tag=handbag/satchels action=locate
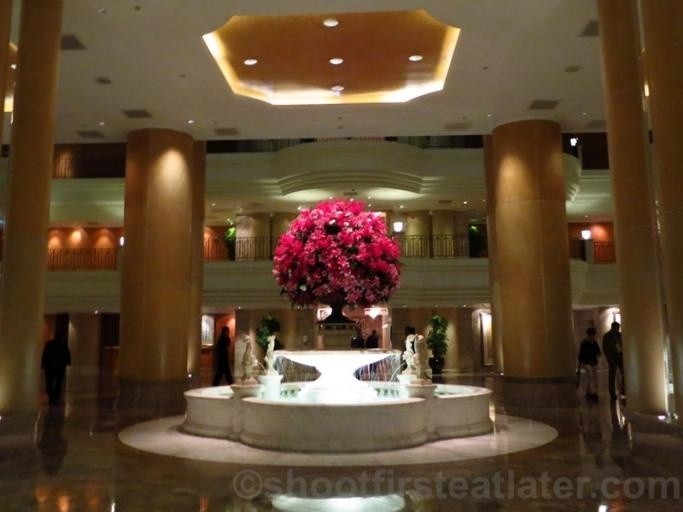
[572,371,581,388]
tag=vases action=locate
[314,302,357,349]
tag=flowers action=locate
[269,198,398,312]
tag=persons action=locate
[577,327,602,401]
[403,327,416,370]
[40,325,72,406]
[601,320,626,402]
[210,325,233,387]
[263,330,285,356]
[350,327,379,348]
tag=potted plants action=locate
[425,313,448,375]
